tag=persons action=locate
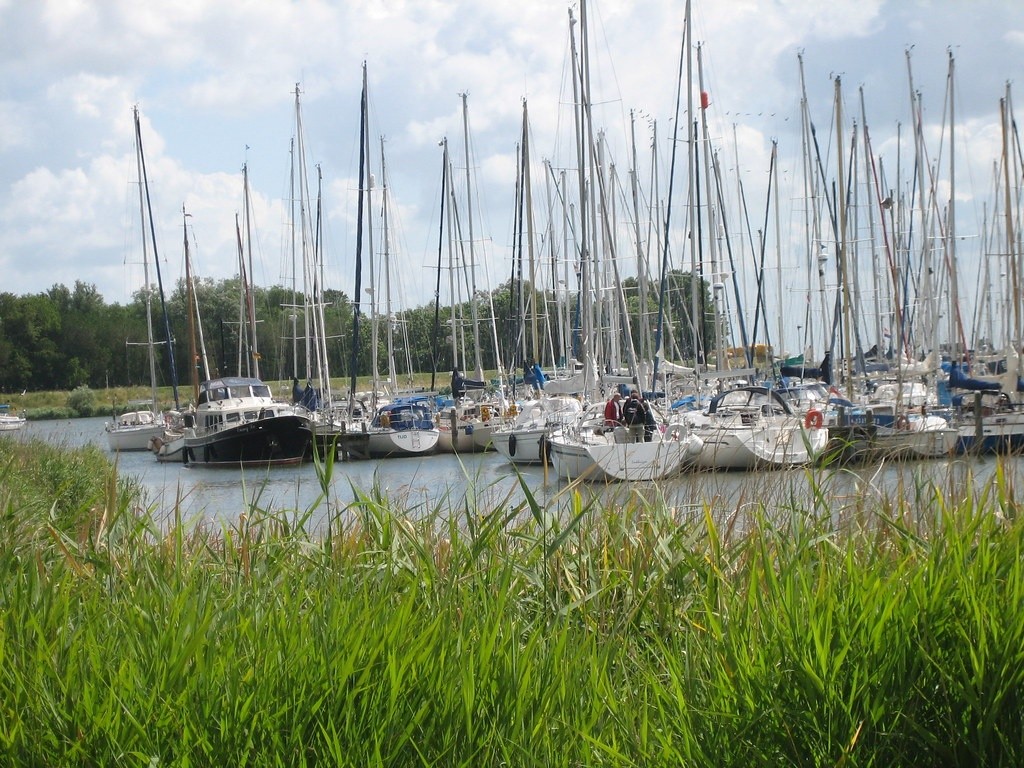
[604,393,623,427]
[623,389,657,444]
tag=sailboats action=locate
[103,0,1024,483]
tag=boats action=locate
[0,403,28,431]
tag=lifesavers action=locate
[804,410,822,430]
